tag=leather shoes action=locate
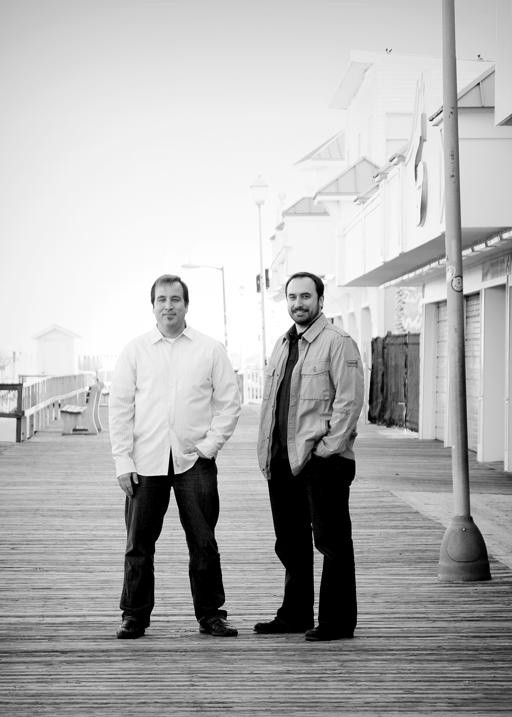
[304,624,354,641]
[199,617,238,637]
[116,618,148,639]
[253,619,314,634]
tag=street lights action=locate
[248,169,270,395]
[179,263,228,361]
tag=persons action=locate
[250,271,366,643]
[104,272,242,641]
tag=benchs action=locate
[58,381,104,437]
